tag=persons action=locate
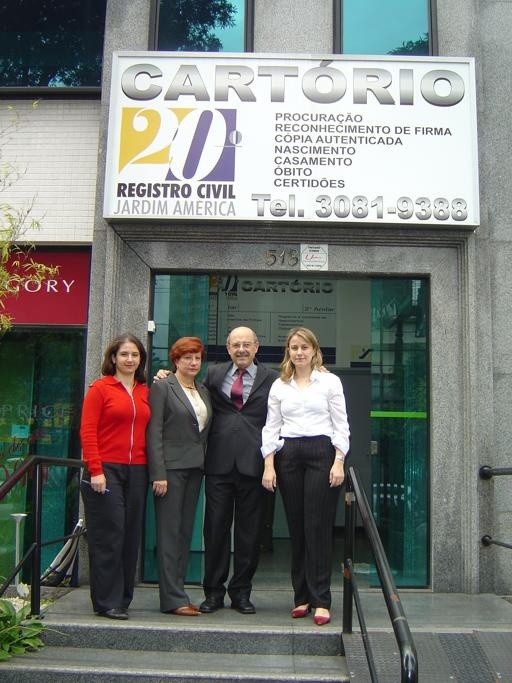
[260,324,352,626]
[152,324,331,616]
[77,331,152,620]
[145,335,214,617]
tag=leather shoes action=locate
[292,604,330,625]
[174,597,224,615]
[231,599,255,614]
[107,608,128,619]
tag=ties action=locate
[231,369,246,410]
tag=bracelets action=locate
[335,455,344,462]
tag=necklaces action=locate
[179,378,196,389]
